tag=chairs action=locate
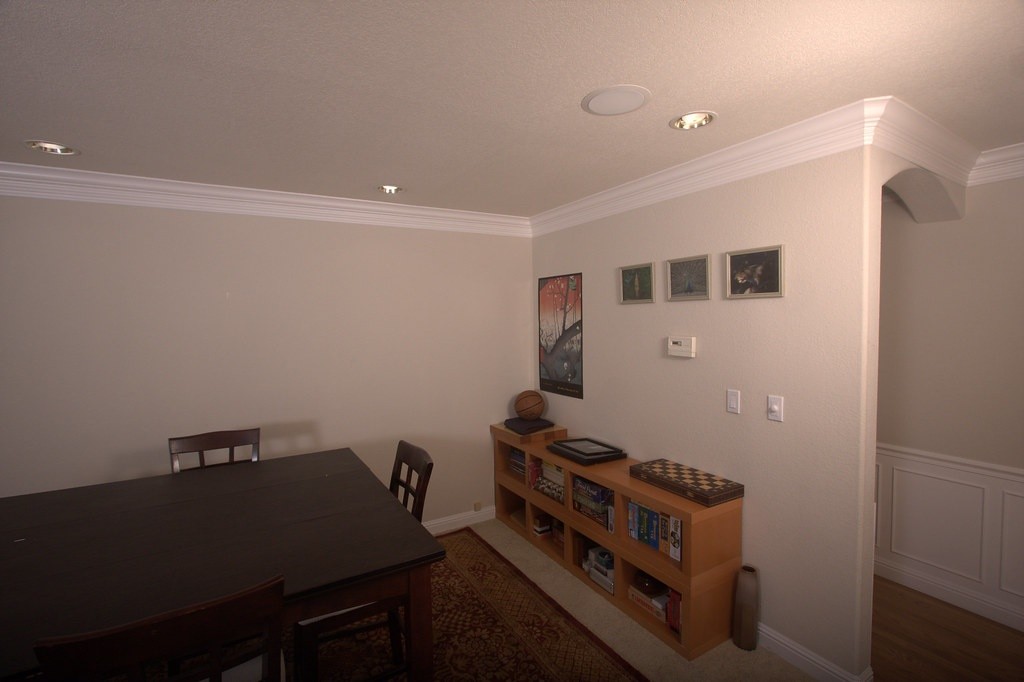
[33,574,287,682]
[168,428,261,473]
[295,442,433,682]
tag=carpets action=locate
[280,525,650,682]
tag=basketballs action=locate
[514,390,545,421]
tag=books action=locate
[627,576,681,636]
[573,535,615,595]
[529,459,564,502]
[624,497,682,561]
[572,476,613,528]
[533,516,564,549]
[510,447,525,475]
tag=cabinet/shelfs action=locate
[490,420,745,661]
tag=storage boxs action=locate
[628,503,639,540]
[648,510,659,550]
[638,507,650,545]
[659,511,671,556]
[589,568,614,595]
[670,516,682,562]
[628,583,668,624]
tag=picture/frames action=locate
[725,243,786,299]
[538,272,584,400]
[667,253,713,301]
[618,262,656,304]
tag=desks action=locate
[0,447,447,682]
